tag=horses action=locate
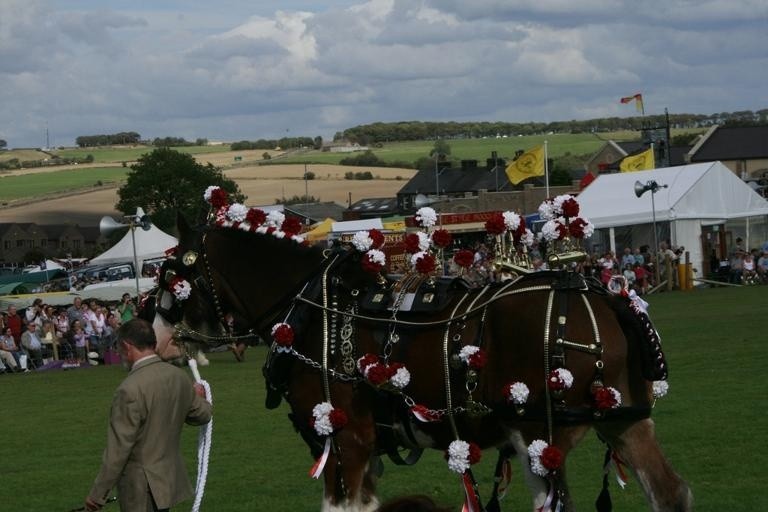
[149,210,693,512]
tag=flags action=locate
[619,148,654,173]
[505,145,546,186]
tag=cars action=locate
[0,265,41,275]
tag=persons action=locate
[0,264,251,373]
[84,318,213,512]
